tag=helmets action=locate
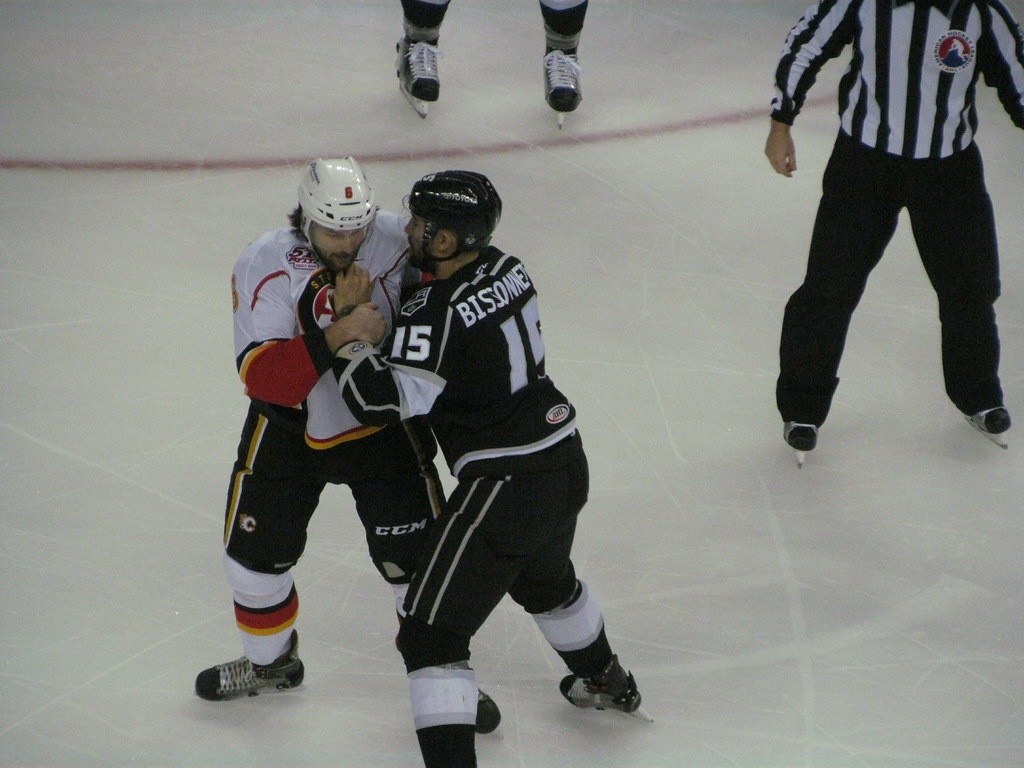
[296,153,377,228]
[410,170,504,235]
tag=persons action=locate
[396,0,588,130]
[196,156,654,768]
[765,0,1024,469]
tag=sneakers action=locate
[542,50,583,131]
[783,422,818,466]
[397,36,441,120]
[476,690,502,736]
[965,407,1011,449]
[559,654,654,723]
[194,628,306,702]
